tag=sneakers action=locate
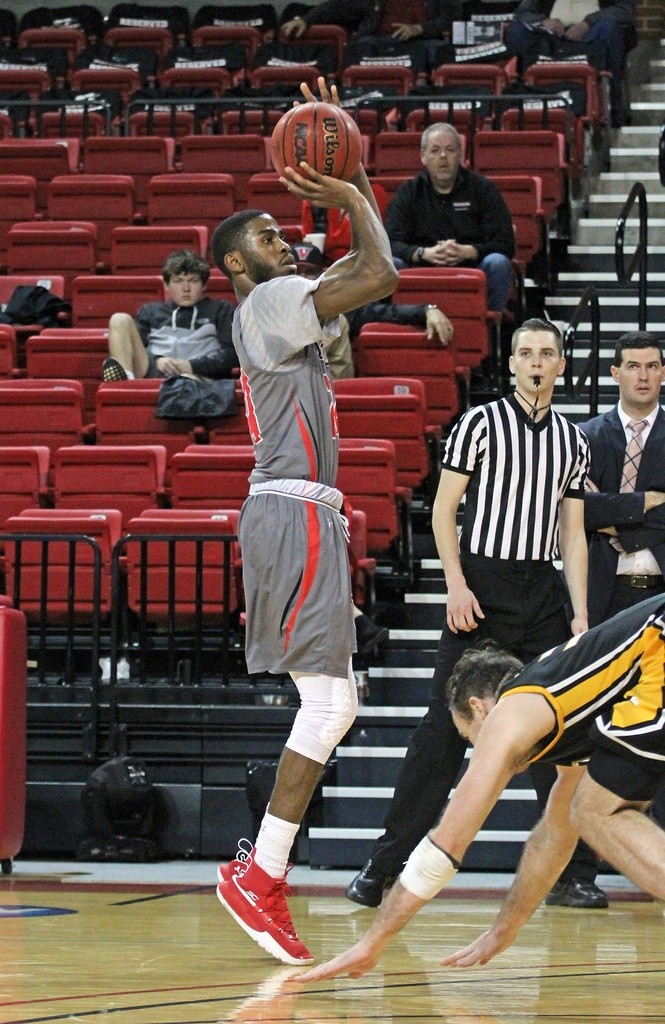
[102,358,129,383]
[214,859,315,968]
[217,846,259,880]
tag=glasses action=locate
[293,268,319,275]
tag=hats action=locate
[290,243,324,270]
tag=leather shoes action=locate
[544,877,610,908]
[345,859,385,908]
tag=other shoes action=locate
[354,613,389,655]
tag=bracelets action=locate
[425,304,438,312]
[418,246,424,261]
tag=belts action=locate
[615,574,664,590]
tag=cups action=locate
[305,233,326,255]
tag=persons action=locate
[212,156,400,968]
[385,122,516,313]
[102,248,240,383]
[572,332,665,633]
[288,242,455,379]
[345,314,611,908]
[277,0,637,86]
[292,593,665,983]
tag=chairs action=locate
[0,0,611,674]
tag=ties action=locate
[619,418,650,495]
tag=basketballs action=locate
[269,100,362,189]
